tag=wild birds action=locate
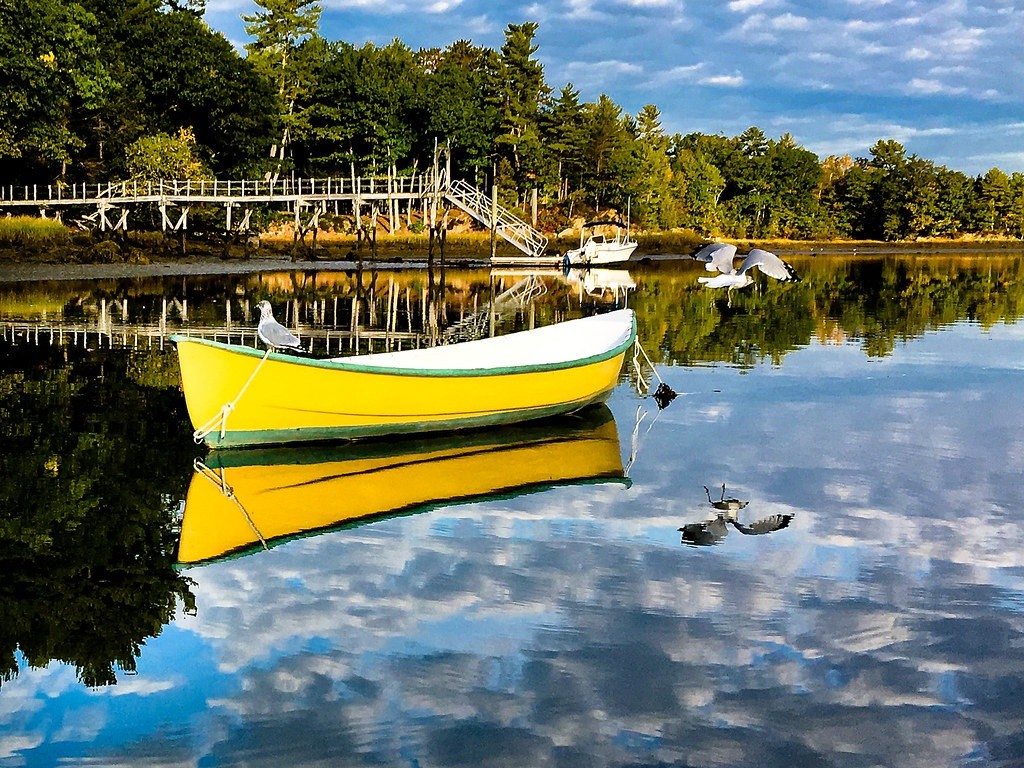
[254,299,308,354]
[675,482,798,549]
[689,240,804,312]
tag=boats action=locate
[169,303,639,450]
[566,194,639,266]
[567,270,635,311]
[173,403,634,576]
